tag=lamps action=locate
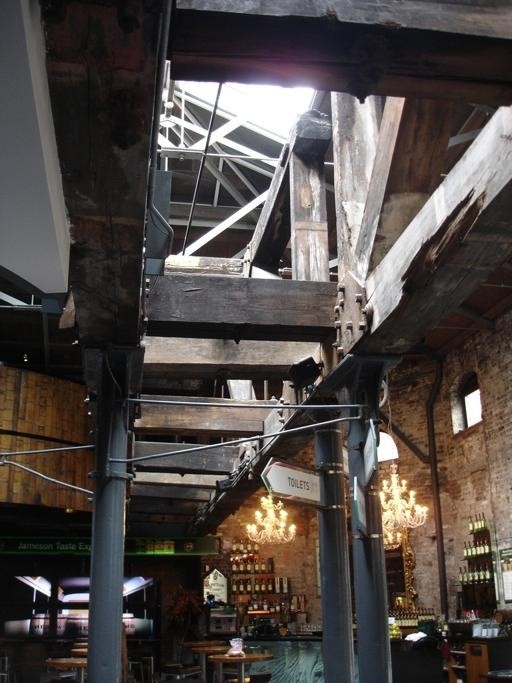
[246,492,297,546]
[378,372,429,551]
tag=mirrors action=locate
[382,524,418,611]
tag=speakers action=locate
[289,356,321,391]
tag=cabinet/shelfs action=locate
[229,551,294,615]
[450,529,493,587]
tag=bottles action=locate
[231,577,274,594]
[231,534,259,553]
[231,556,267,574]
[388,605,437,629]
[463,537,491,558]
[468,511,487,533]
[458,563,493,584]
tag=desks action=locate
[42,633,276,683]
